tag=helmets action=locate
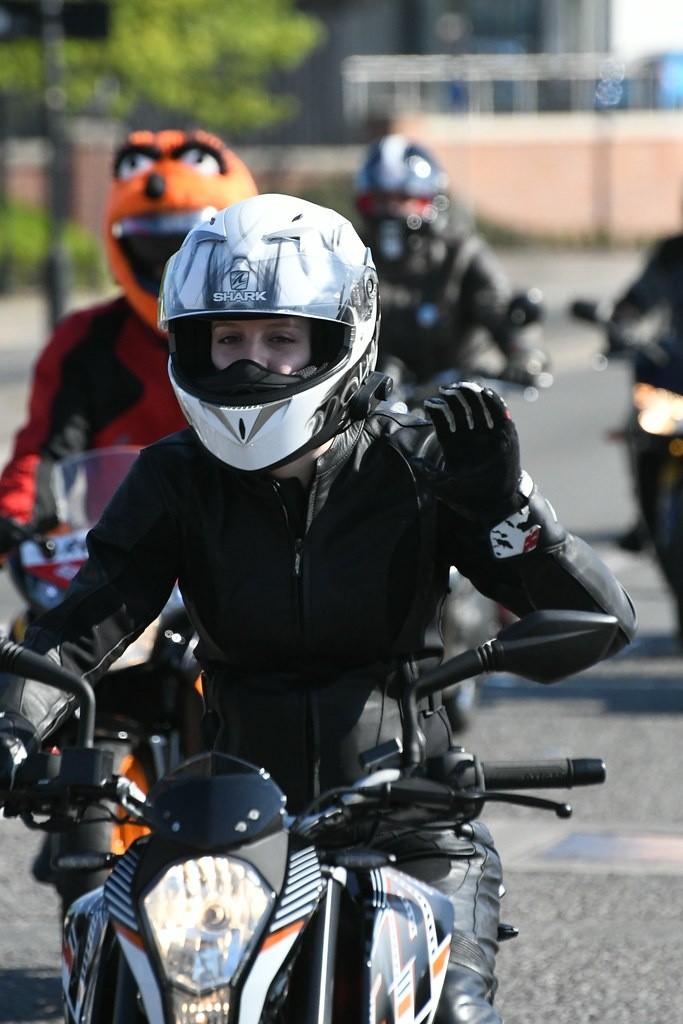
[156,194,393,477]
[354,133,448,285]
[112,215,210,295]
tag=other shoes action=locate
[618,520,651,551]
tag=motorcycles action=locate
[351,289,549,730]
[1,447,215,799]
[2,543,635,1024]
[565,293,683,638]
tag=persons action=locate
[606,234,683,551]
[0,130,258,617]
[352,135,548,625]
[0,193,636,1024]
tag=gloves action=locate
[0,710,41,808]
[499,355,533,394]
[409,384,566,529]
